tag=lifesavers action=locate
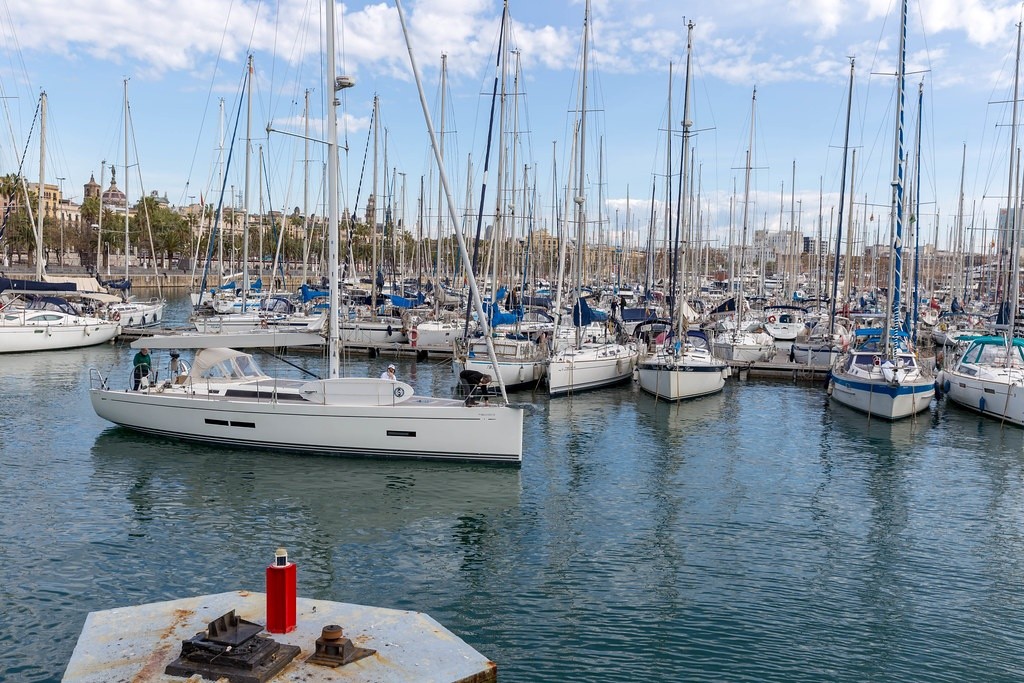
[768,315,776,323]
[112,310,122,322]
[261,319,267,326]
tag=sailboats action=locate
[937,169,1024,425]
[88,0,522,471]
[828,0,937,421]
[638,16,732,400]
[543,0,647,394]
[452,0,549,391]
[0,0,1024,373]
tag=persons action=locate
[380,364,396,381]
[132,347,154,391]
[460,370,493,407]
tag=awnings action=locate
[0,274,124,305]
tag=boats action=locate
[0,291,122,353]
